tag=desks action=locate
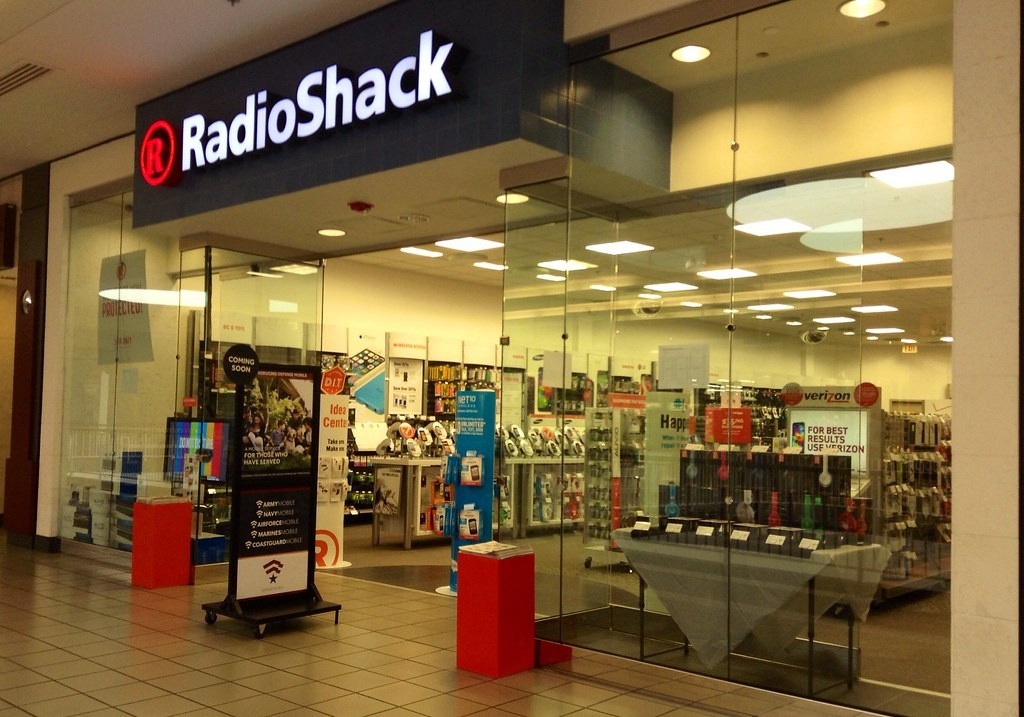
[610,527,893,697]
[369,457,585,551]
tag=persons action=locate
[241,409,312,455]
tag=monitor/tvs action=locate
[163,417,234,486]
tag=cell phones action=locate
[349,346,387,395]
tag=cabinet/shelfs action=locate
[344,451,377,525]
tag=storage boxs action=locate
[192,532,225,564]
[59,473,171,552]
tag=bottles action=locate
[686,451,698,478]
[840,498,856,531]
[819,454,832,487]
[718,451,729,479]
[768,491,781,526]
[856,504,868,537]
[801,495,814,529]
[665,484,680,517]
